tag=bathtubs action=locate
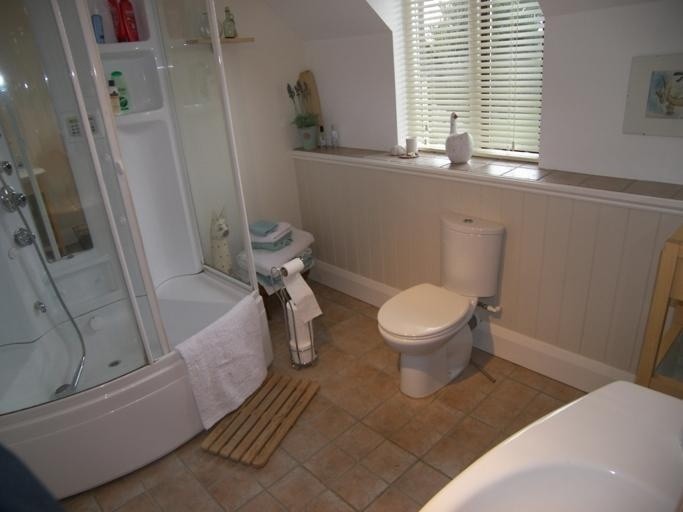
[421,380,682,512]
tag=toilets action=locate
[377,213,505,398]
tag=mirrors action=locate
[0,0,96,263]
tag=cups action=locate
[406,139,418,153]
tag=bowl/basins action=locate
[391,145,405,155]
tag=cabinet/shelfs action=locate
[635,224,683,399]
[79,0,169,129]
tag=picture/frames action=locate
[620,53,683,136]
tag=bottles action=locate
[91,9,104,42]
[223,5,237,38]
[319,124,337,149]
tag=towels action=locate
[249,219,316,297]
[176,295,267,430]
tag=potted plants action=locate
[287,81,322,151]
